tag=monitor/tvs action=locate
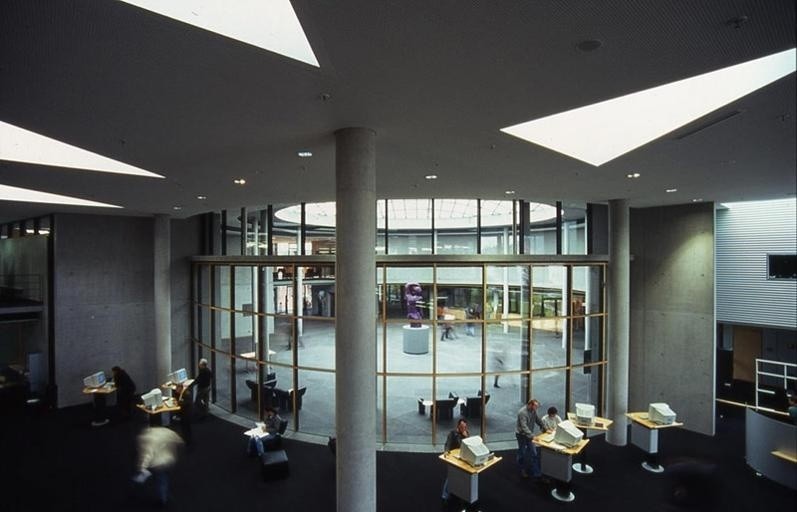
[555,419,585,448]
[141,387,164,409]
[459,435,489,467]
[648,402,677,424]
[167,367,189,384]
[83,370,107,388]
[575,402,595,424]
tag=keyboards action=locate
[640,415,648,419]
[164,400,175,408]
[541,431,556,442]
[453,454,461,459]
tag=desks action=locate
[438,411,685,505]
[81,378,185,427]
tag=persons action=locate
[438,418,470,507]
[438,305,452,340]
[464,306,476,337]
[513,399,546,480]
[110,365,136,419]
[243,406,283,457]
[191,358,212,408]
[404,281,425,328]
[136,422,186,506]
[540,406,561,432]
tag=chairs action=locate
[419,390,490,423]
[245,377,306,413]
[263,418,288,449]
